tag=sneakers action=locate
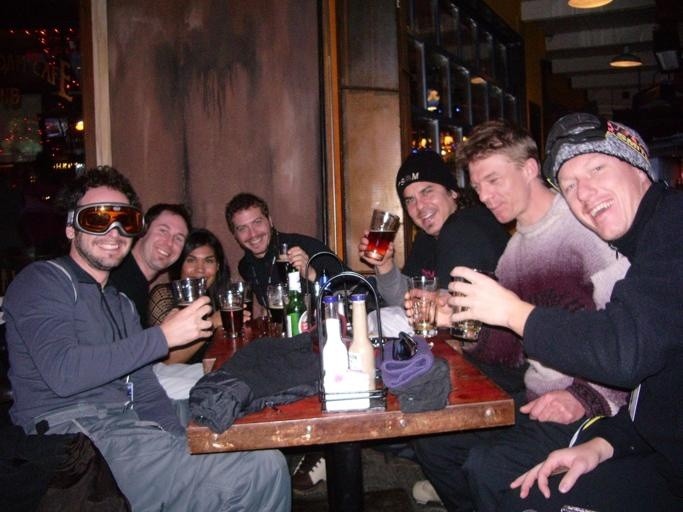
[412,479,444,508]
[287,452,327,497]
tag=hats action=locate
[546,119,653,190]
[395,148,459,191]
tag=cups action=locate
[218,290,243,338]
[265,284,289,325]
[450,268,481,340]
[407,277,440,337]
[362,208,400,266]
[276,244,289,282]
[172,276,207,312]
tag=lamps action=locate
[566,0,614,9]
[607,46,646,68]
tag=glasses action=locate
[391,329,417,360]
[54,201,146,237]
[543,111,651,184]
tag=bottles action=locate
[348,292,376,376]
[319,295,349,379]
[315,268,330,316]
[284,266,310,340]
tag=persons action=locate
[227,194,358,322]
[359,146,510,312]
[2,166,294,512]
[110,205,191,328]
[403,120,641,485]
[151,229,252,339]
[449,117,683,512]
